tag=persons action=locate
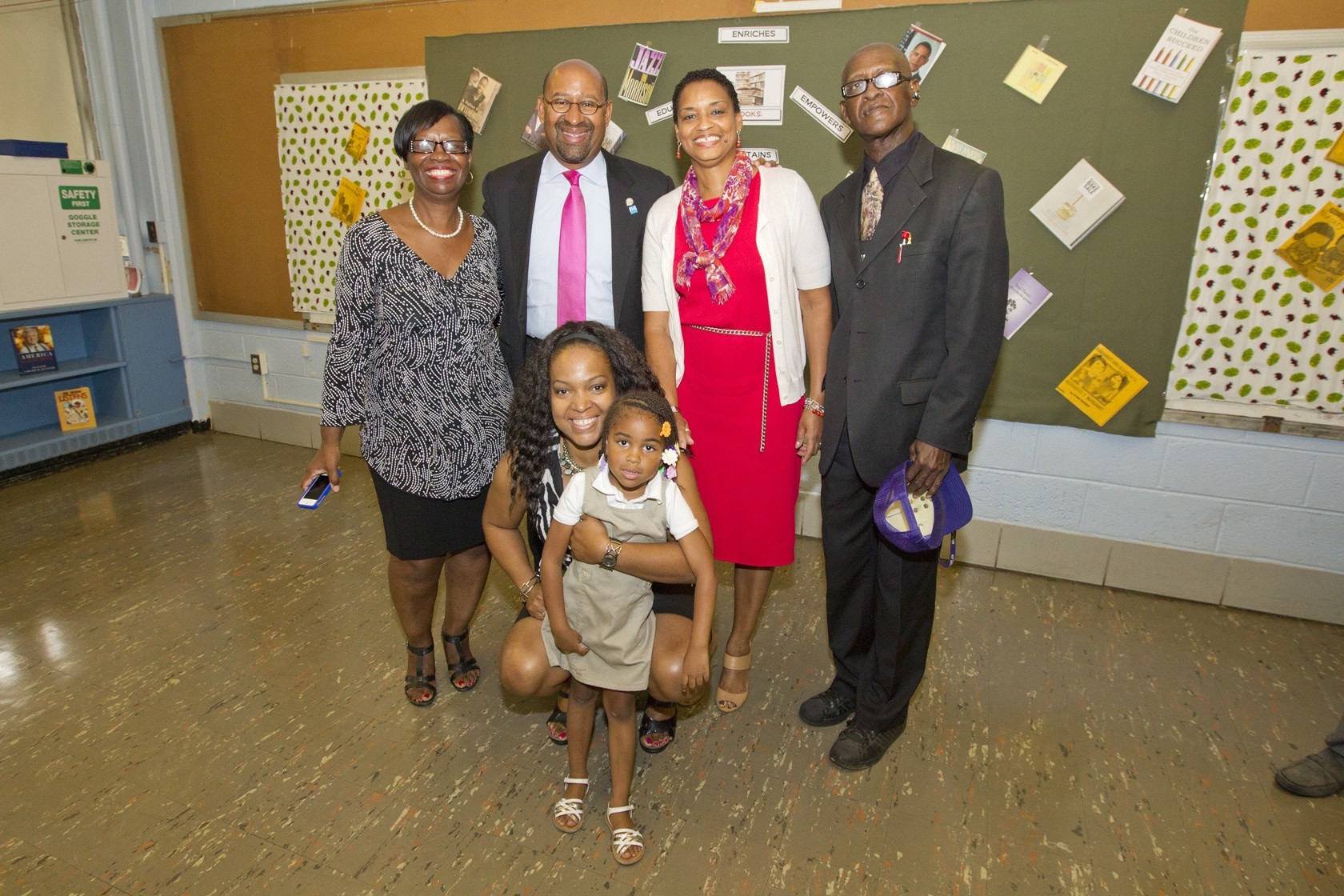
[795,44,1008,771]
[539,386,717,866]
[908,42,932,82]
[476,321,715,752]
[460,76,490,115]
[1274,713,1344,799]
[481,57,782,384]
[638,68,834,713]
[18,327,52,354]
[301,99,515,707]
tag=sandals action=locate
[553,774,589,834]
[718,638,753,713]
[440,623,481,692]
[603,800,645,865]
[640,696,678,754]
[404,638,435,706]
[680,634,713,707]
[546,690,571,746]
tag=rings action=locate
[685,428,691,434]
[819,443,821,446]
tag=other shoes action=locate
[1274,749,1344,796]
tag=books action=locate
[54,386,98,432]
[10,323,59,376]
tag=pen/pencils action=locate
[897,231,911,264]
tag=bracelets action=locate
[518,574,541,605]
[599,537,623,572]
[670,405,680,413]
[802,397,826,418]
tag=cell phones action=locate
[298,470,342,510]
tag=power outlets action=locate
[251,352,268,375]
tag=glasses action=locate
[841,71,911,98]
[408,139,469,154]
[542,96,607,115]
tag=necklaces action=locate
[409,196,464,239]
[557,435,587,478]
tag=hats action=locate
[872,459,973,552]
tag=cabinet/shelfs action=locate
[0,292,193,471]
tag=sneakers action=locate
[800,690,856,727]
[829,714,908,769]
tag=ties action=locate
[557,172,587,328]
[861,167,884,243]
